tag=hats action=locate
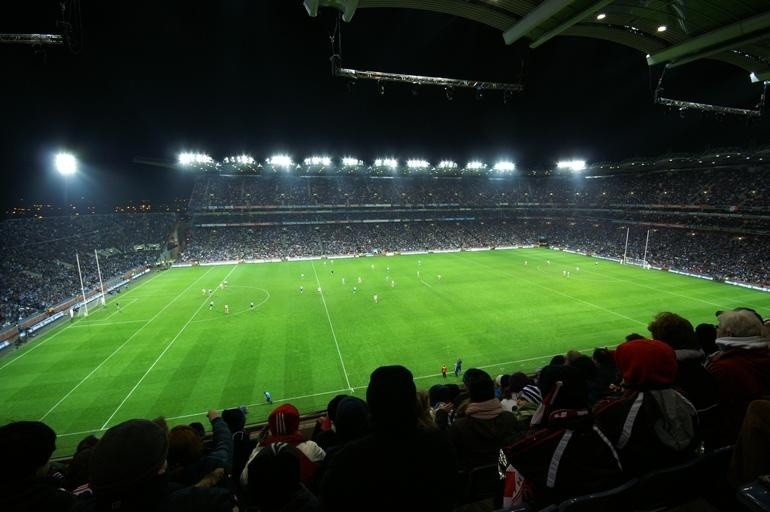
[429,384,459,406]
[73,436,97,472]
[695,323,717,343]
[616,339,677,391]
[539,366,591,412]
[222,410,245,435]
[0,421,56,480]
[268,405,298,442]
[249,442,300,491]
[518,384,543,407]
[463,369,495,401]
[91,419,169,489]
[168,425,200,484]
[328,366,418,437]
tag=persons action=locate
[250,301,255,312]
[171,168,770,296]
[223,304,229,314]
[209,299,214,310]
[0,207,169,309]
[374,293,378,304]
[0,307,769,510]
